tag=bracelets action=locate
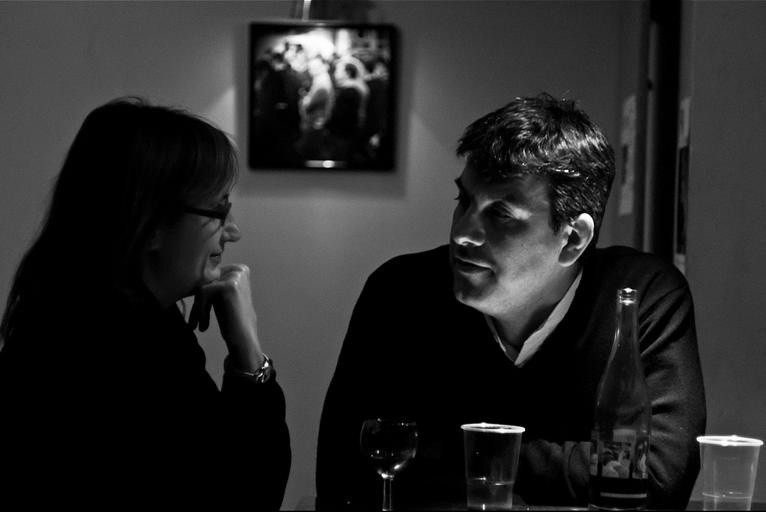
[222,349,273,384]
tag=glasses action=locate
[180,202,233,226]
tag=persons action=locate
[2,99,293,510]
[313,92,707,512]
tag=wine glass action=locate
[359,415,421,512]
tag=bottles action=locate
[588,289,652,511]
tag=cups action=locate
[696,435,763,511]
[461,423,525,511]
